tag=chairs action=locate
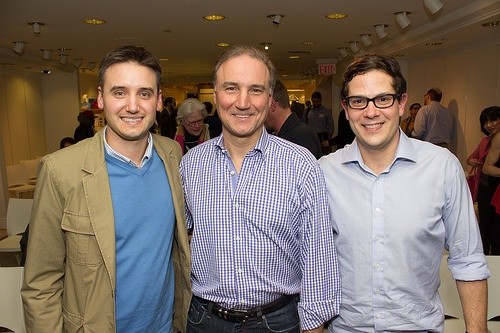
[0,159,40,333]
[438,254,500,333]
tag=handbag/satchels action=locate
[466,166,477,204]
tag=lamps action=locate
[86,61,96,71]
[12,41,24,55]
[337,47,350,59]
[394,11,412,31]
[74,59,82,68]
[31,22,41,34]
[423,0,444,16]
[270,14,282,25]
[41,48,52,61]
[360,33,372,47]
[374,23,388,40]
[58,48,70,65]
[348,40,361,54]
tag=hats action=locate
[77,111,94,124]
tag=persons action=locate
[302,91,335,156]
[73,109,96,143]
[158,96,177,140]
[176,45,343,333]
[322,90,356,150]
[411,87,454,149]
[263,79,323,160]
[202,101,223,139]
[316,53,491,333]
[482,132,500,255]
[306,101,312,110]
[59,137,75,149]
[400,103,422,138]
[466,106,500,256]
[19,43,193,333]
[173,97,210,157]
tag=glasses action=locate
[344,93,404,110]
[183,117,205,126]
[424,93,430,97]
[413,106,420,110]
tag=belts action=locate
[194,293,299,323]
